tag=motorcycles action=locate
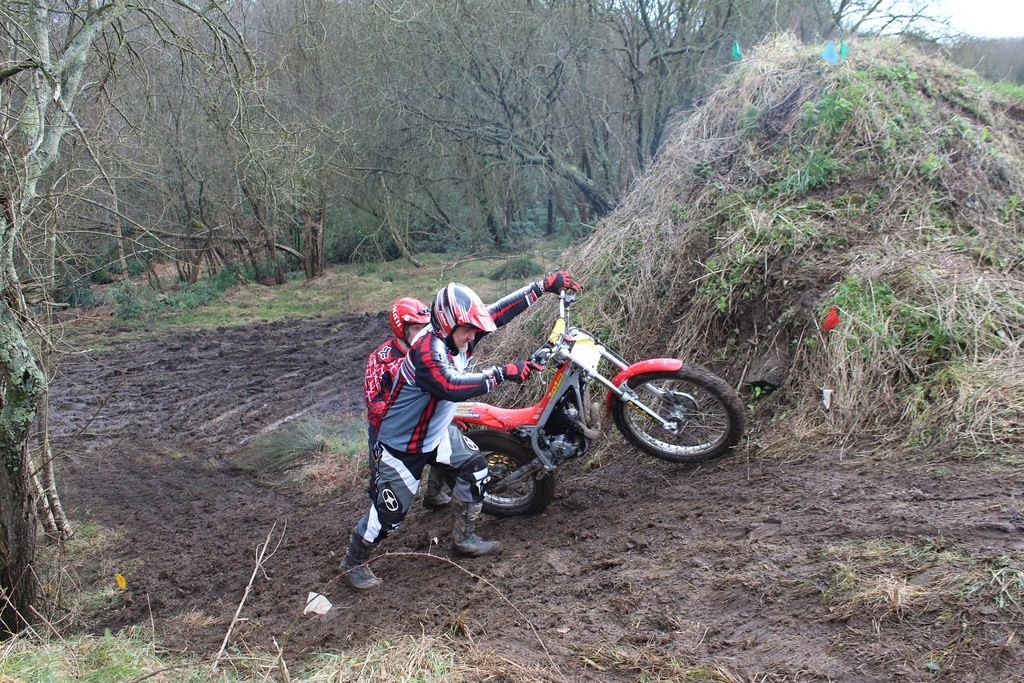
[443,268,749,517]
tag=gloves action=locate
[543,269,581,295]
[502,361,545,384]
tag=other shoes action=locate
[422,490,452,509]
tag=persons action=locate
[364,296,452,534]
[339,269,582,591]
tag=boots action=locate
[450,493,503,558]
[339,527,380,593]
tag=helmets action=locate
[390,297,431,340]
[431,282,497,339]
